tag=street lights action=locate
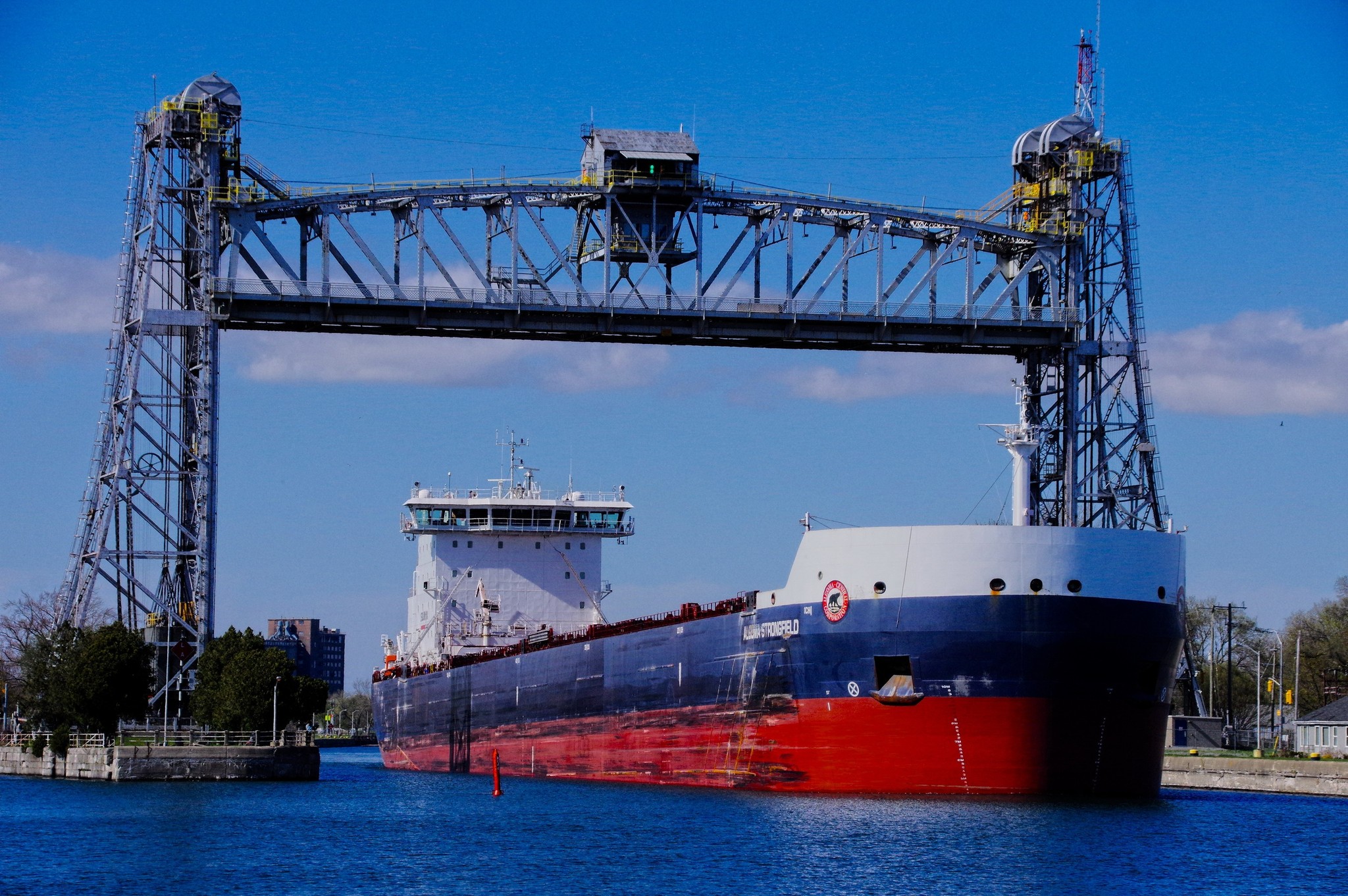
[338,709,347,735]
[1234,643,1260,749]
[273,677,282,741]
[1269,630,1282,749]
[351,710,359,735]
[327,708,335,734]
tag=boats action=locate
[372,374,1187,807]
